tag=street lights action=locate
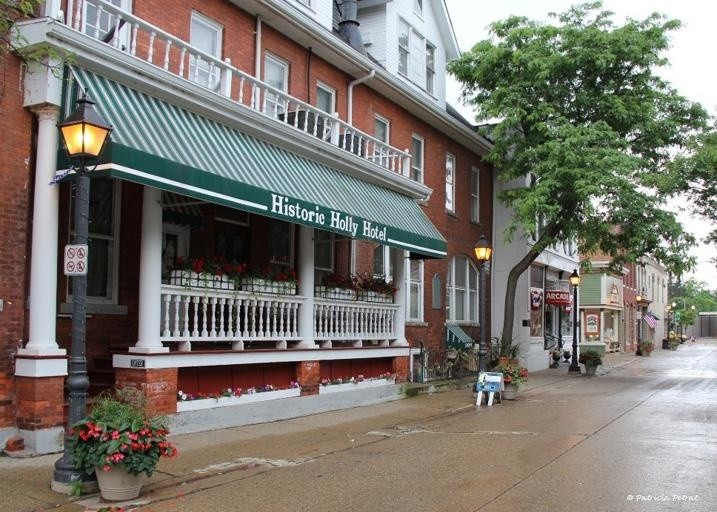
[691,304,695,336]
[671,301,676,324]
[568,269,581,371]
[51,86,115,483]
[666,304,671,339]
[472,233,493,392]
[635,292,642,356]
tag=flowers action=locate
[495,365,528,384]
[64,387,177,478]
[178,382,304,402]
[319,372,396,386]
[170,255,397,304]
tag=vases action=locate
[502,383,517,400]
[93,464,142,502]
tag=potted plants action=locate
[639,341,654,356]
[509,344,520,370]
[580,351,602,374]
[498,344,511,368]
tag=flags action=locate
[643,311,658,329]
